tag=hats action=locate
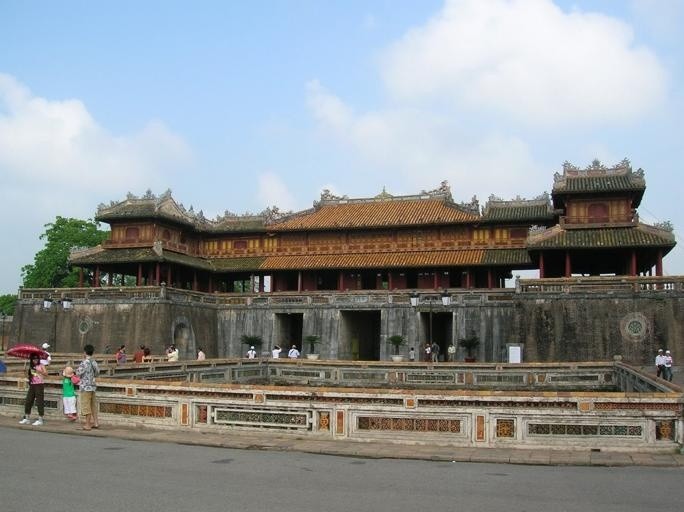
[250,346,255,350]
[658,349,663,353]
[292,345,296,349]
[425,344,431,347]
[62,366,74,377]
[42,342,51,350]
[666,350,670,354]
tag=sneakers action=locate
[67,414,77,421]
[18,418,30,425]
[31,420,43,426]
[76,423,99,430]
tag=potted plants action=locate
[457,335,480,363]
[385,334,408,364]
[304,335,325,361]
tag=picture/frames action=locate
[506,342,524,363]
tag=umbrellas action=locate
[5,344,48,369]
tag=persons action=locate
[245,345,256,360]
[73,344,100,431]
[287,344,300,360]
[195,347,206,360]
[40,343,52,366]
[430,341,439,362]
[407,346,415,361]
[60,365,78,422]
[114,344,179,364]
[654,348,666,379]
[663,350,674,381]
[272,344,281,358]
[423,343,431,361]
[15,353,48,425]
[446,342,455,361]
